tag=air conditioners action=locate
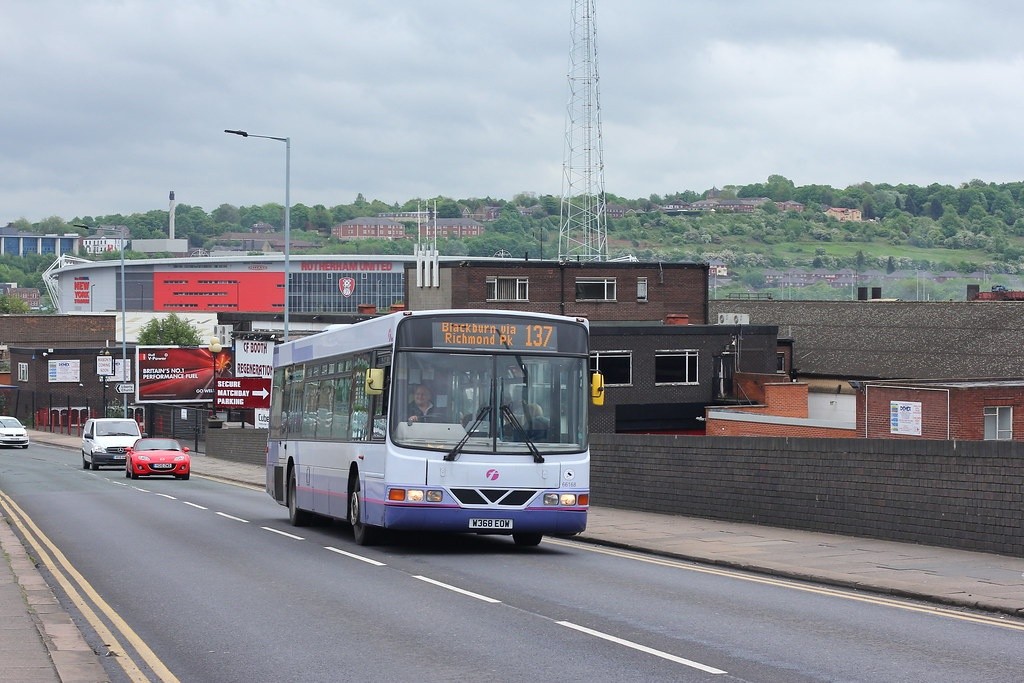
[718,313,749,325]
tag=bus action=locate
[266,312,603,549]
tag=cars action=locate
[281,408,334,430]
[350,412,386,441]
[124,438,191,480]
[0,415,29,449]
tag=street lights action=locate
[915,269,918,300]
[208,337,224,416]
[73,224,128,421]
[712,271,717,300]
[98,348,111,417]
[983,270,985,289]
[223,130,289,342]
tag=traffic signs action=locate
[116,384,135,394]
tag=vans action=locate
[80,419,148,470]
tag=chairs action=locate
[281,408,385,440]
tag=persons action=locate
[407,384,444,423]
[521,404,551,442]
